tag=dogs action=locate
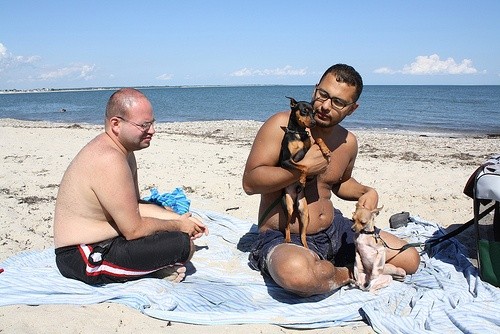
[257,96,331,249]
[351,204,393,293]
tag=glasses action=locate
[115,116,155,129]
[314,88,356,110]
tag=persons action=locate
[54,88,209,287]
[243,63,420,298]
